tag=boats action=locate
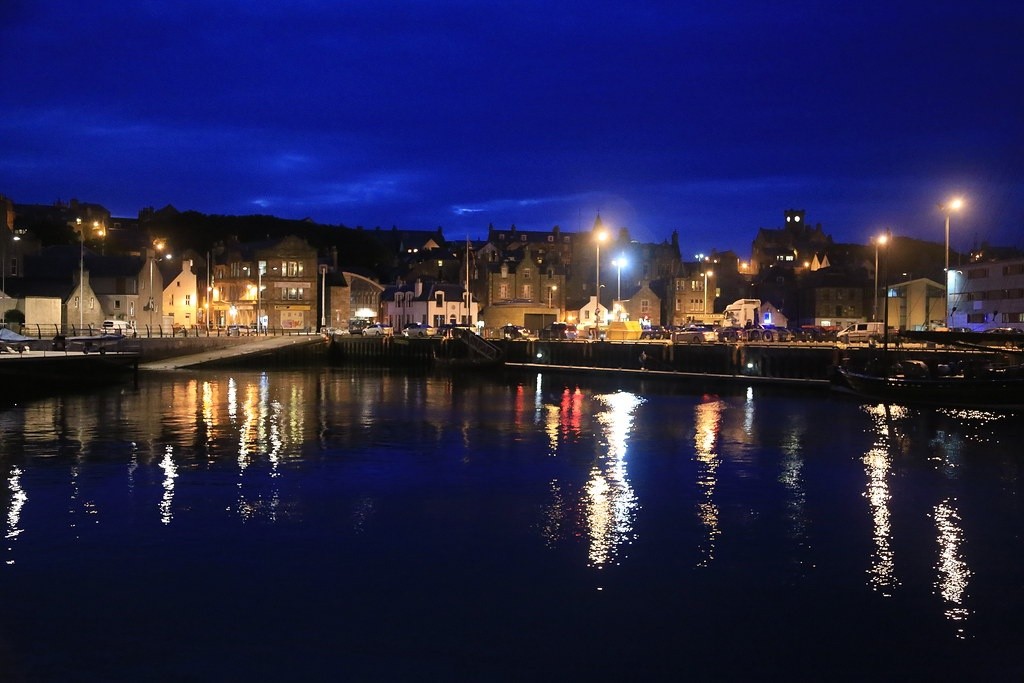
[839,360,1024,409]
[432,346,505,378]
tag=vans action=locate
[101,320,137,338]
[837,322,886,344]
[947,328,971,333]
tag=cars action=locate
[539,322,578,341]
[888,326,899,334]
[362,323,394,336]
[641,325,661,339]
[670,325,717,345]
[350,320,370,335]
[226,325,256,336]
[500,326,531,342]
[713,326,747,342]
[402,324,438,336]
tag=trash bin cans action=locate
[231,328,239,337]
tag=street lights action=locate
[595,231,609,344]
[612,255,627,322]
[873,235,887,322]
[2,234,21,328]
[242,265,277,336]
[700,271,713,321]
[937,199,966,327]
[149,255,172,338]
[80,229,103,338]
[549,286,558,309]
